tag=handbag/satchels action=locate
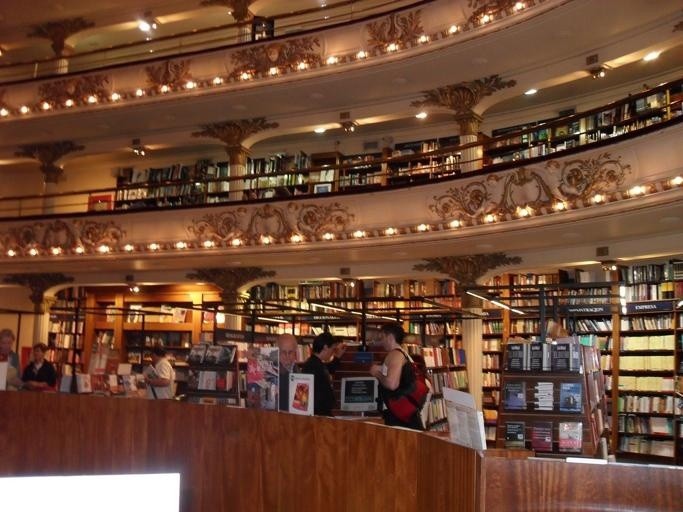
[377,349,430,423]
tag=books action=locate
[551,90,683,154]
[242,153,383,202]
[483,259,683,457]
[202,279,409,407]
[116,159,233,211]
[48,302,192,399]
[409,279,469,432]
[493,120,551,165]
[399,140,461,185]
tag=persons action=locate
[371,324,433,430]
[0,328,21,391]
[266,331,347,418]
[22,341,57,390]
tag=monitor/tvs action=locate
[340,377,380,413]
[0,471,188,511]
